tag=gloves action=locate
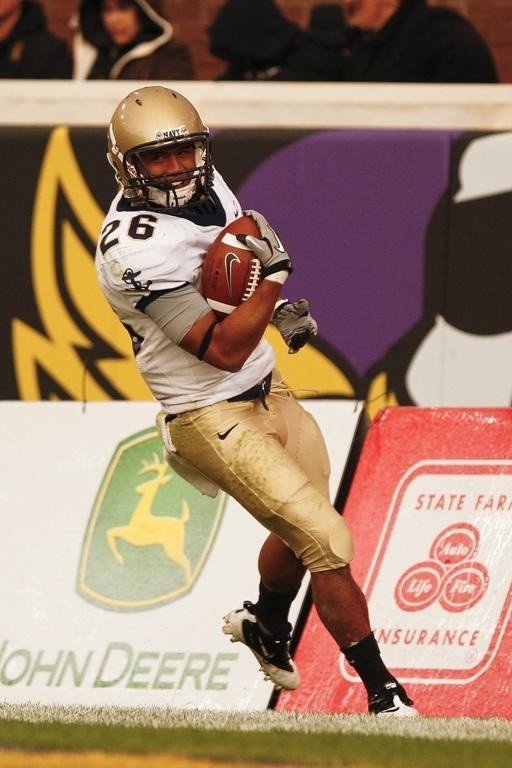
[235,209,294,276]
[274,298,318,353]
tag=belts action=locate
[228,372,273,410]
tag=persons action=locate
[92,83,421,722]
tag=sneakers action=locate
[223,607,300,691]
[367,677,418,719]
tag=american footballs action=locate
[200,216,262,322]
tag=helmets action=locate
[105,86,209,199]
